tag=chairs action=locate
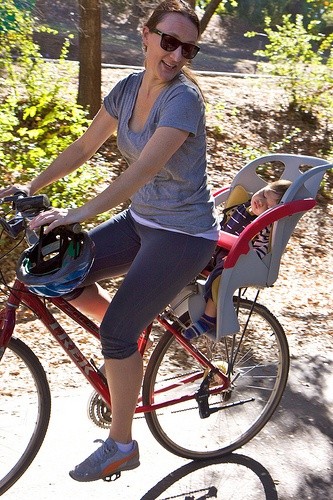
[172,154,332,341]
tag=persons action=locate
[0,0,221,482]
[182,180,295,339]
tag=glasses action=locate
[150,27,200,59]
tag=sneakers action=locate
[97,332,153,377]
[69,438,140,483]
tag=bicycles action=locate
[0,186,290,499]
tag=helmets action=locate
[16,223,96,298]
